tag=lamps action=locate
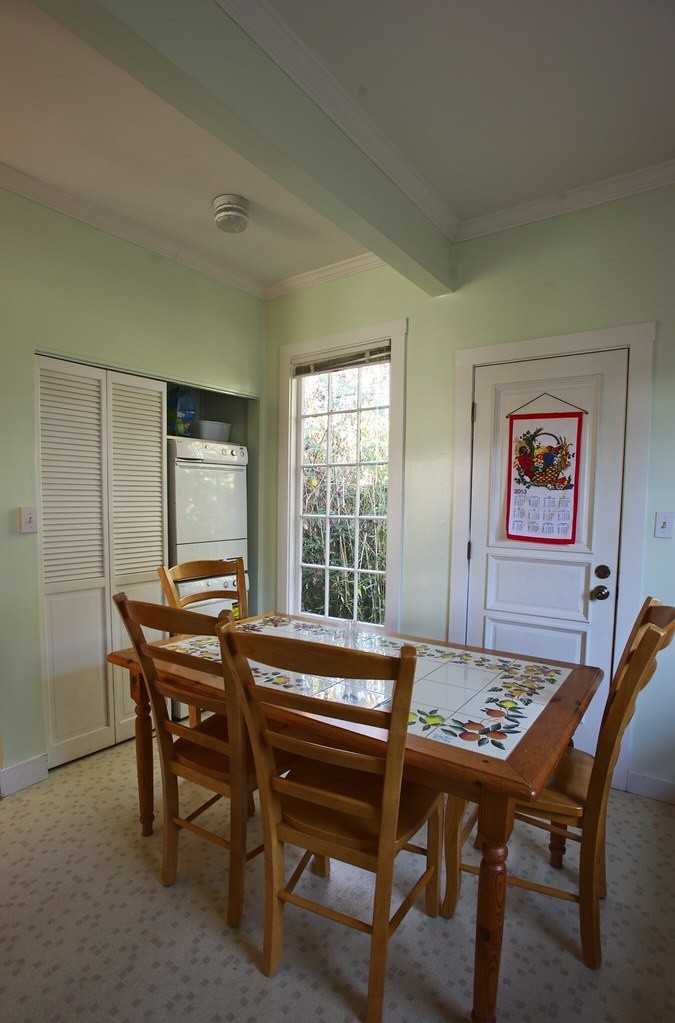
[213,194,250,234]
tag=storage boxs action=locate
[188,420,232,441]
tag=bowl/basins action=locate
[192,420,232,442]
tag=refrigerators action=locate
[168,439,249,719]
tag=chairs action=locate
[439,595,675,969]
[156,557,246,726]
[213,618,443,1023]
[112,590,312,930]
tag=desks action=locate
[107,612,604,1023]
